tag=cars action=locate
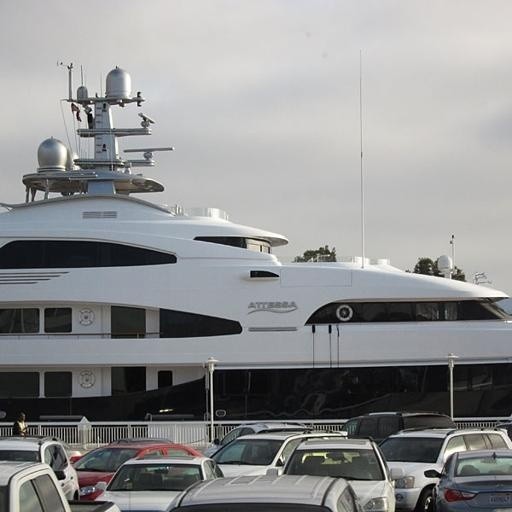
[72,435,225,512]
[424,448,512,511]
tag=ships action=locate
[0,61,510,422]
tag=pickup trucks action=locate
[0,459,120,512]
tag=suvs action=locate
[375,426,511,511]
[0,433,81,504]
[166,407,458,512]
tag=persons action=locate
[12,412,30,437]
